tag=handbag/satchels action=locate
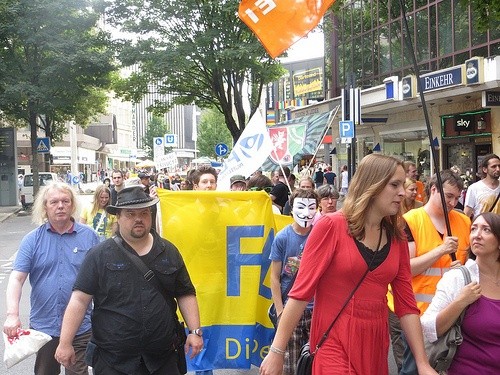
[179,321,187,375]
[295,341,314,375]
[2,327,52,370]
[400,266,474,375]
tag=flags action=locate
[256,105,340,172]
[238,0,337,60]
[215,108,275,191]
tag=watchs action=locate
[188,329,203,336]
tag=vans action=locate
[18,171,58,210]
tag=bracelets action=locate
[270,345,285,356]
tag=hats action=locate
[105,187,159,215]
[138,173,151,179]
[230,175,246,188]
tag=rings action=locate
[200,349,204,351]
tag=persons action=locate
[78,184,118,246]
[73,152,500,375]
[57,186,205,375]
[3,177,102,375]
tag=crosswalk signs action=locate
[36,137,50,153]
